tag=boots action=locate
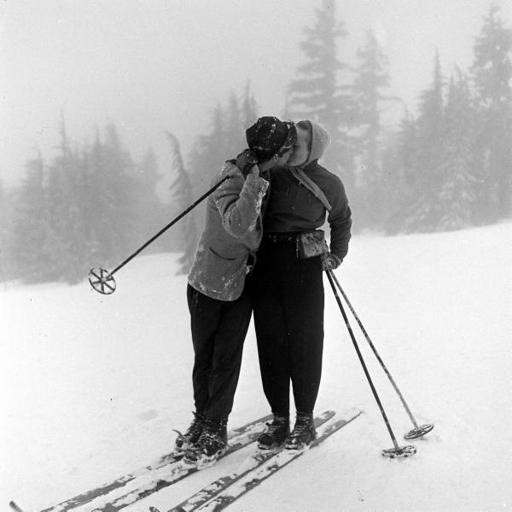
[258,412,317,450]
[173,411,227,461]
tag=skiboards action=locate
[11,414,273,512]
[150,410,366,512]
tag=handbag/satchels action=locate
[297,230,328,259]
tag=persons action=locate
[238,120,353,449]
[175,115,298,463]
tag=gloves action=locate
[322,254,341,270]
[236,149,257,175]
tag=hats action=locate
[300,120,328,168]
[246,116,297,164]
